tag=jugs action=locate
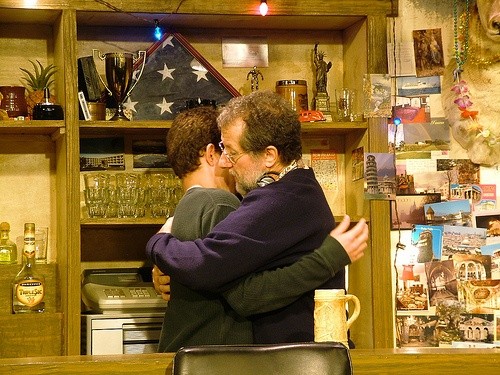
[0,85,29,119]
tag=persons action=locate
[156,106,369,351]
[314,42,332,92]
[247,66,265,92]
[147,90,355,348]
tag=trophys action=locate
[92,49,146,122]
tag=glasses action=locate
[218,141,255,165]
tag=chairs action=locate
[174,342,352,375]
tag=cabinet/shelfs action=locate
[0,0,398,375]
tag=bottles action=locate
[10,223,47,315]
[276,80,308,115]
[0,221,18,264]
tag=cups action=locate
[335,87,356,122]
[313,289,361,349]
[83,171,184,219]
[34,227,48,264]
